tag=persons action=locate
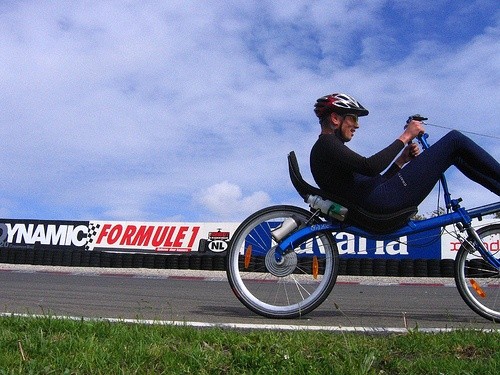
[311,95,500,215]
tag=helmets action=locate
[314,92,369,120]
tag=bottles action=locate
[304,193,348,223]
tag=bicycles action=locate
[227,113,500,322]
